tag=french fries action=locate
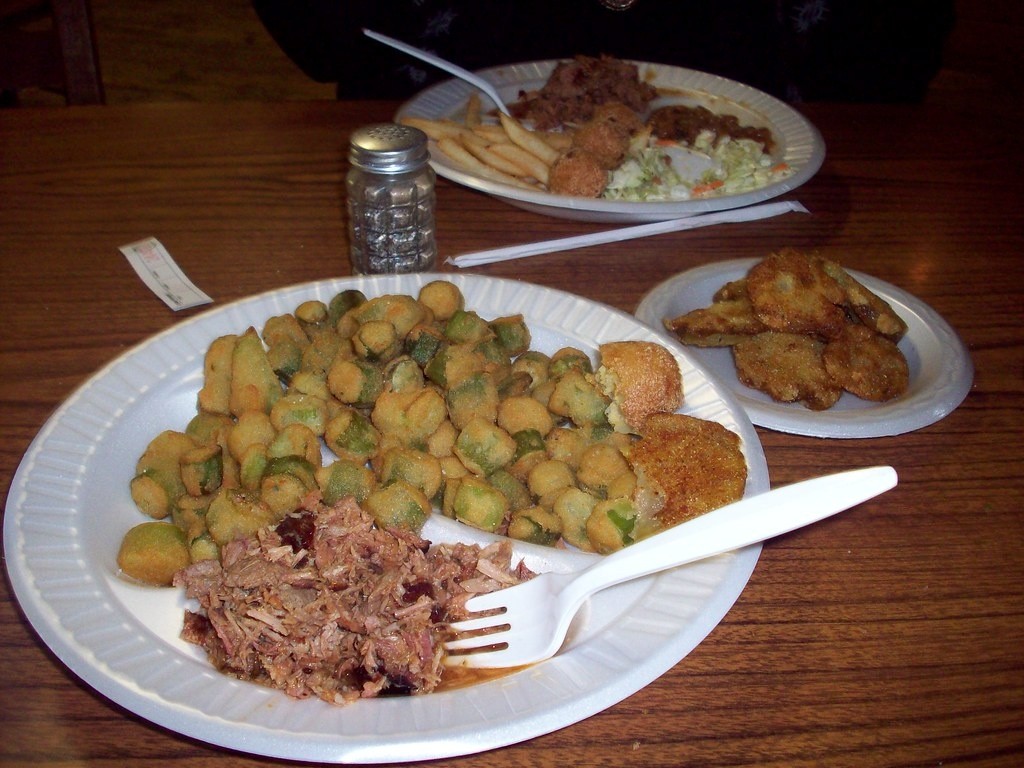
[400,92,582,190]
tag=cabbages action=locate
[605,128,793,201]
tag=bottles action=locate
[345,123,439,274]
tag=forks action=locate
[441,464,897,667]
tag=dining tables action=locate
[1,103,1024,767]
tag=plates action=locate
[393,56,825,222]
[1,271,769,766]
[635,256,974,437]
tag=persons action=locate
[251,0,959,105]
